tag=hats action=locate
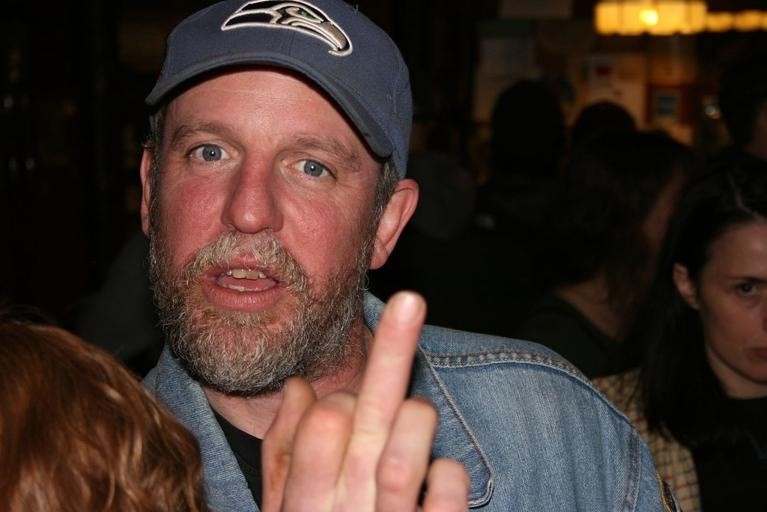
[146,0,414,179]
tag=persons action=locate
[376,57,764,383]
[588,152,765,510]
[138,0,679,511]
[0,319,207,512]
